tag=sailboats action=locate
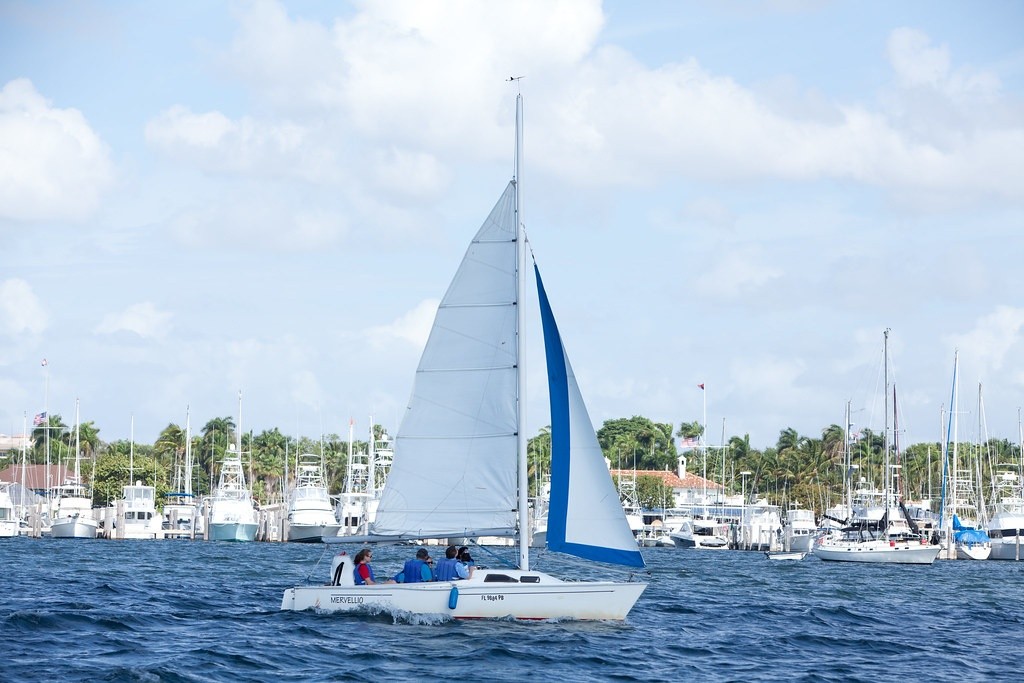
[528,328,1024,564]
[0,359,418,543]
[282,75,649,620]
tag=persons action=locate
[392,545,476,583]
[354,549,397,585]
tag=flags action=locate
[679,434,703,446]
[697,383,705,392]
[34,411,47,426]
[41,358,48,367]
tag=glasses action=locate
[370,555,372,558]
[428,561,433,564]
